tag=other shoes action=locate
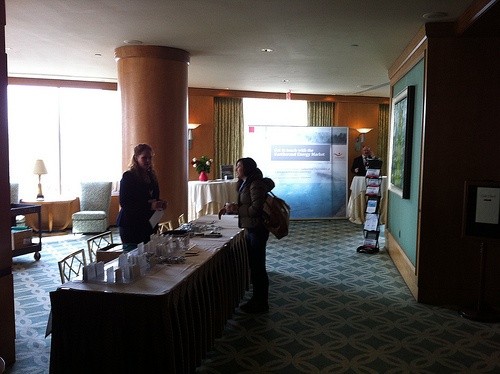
[240,300,270,314]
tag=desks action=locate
[20,196,80,231]
[187,179,239,224]
[45,215,249,374]
[347,175,387,224]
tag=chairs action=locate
[72,182,113,233]
[86,230,113,263]
[58,249,86,285]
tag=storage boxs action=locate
[10,228,32,250]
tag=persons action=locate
[118,144,168,256]
[225,157,270,314]
[352,144,378,176]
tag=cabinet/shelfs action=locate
[10,203,42,261]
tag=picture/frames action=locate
[387,85,414,198]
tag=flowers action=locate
[191,155,214,175]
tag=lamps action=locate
[188,123,202,148]
[355,128,373,143]
[34,160,48,198]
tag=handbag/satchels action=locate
[247,179,290,239]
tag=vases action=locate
[199,171,208,181]
